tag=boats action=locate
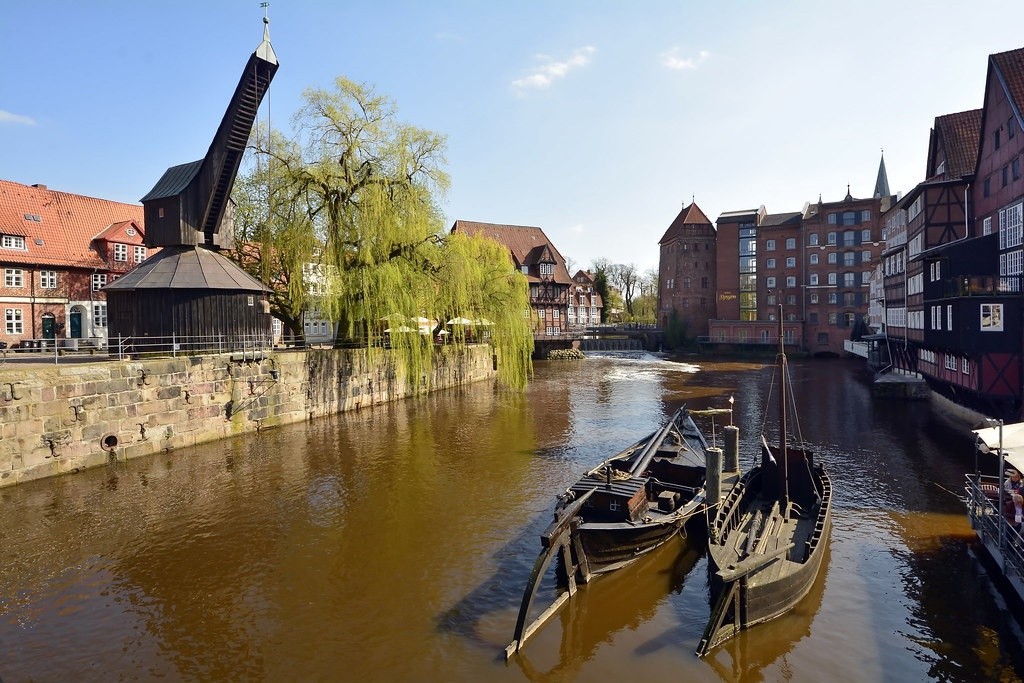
[963,418,1024,612]
[695,304,831,660]
[500,403,740,661]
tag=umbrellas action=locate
[379,313,439,335]
[446,317,496,326]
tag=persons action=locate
[1004,473,1024,558]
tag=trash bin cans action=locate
[32,340,39,353]
[39,340,48,352]
[20,340,33,352]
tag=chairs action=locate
[979,484,999,507]
